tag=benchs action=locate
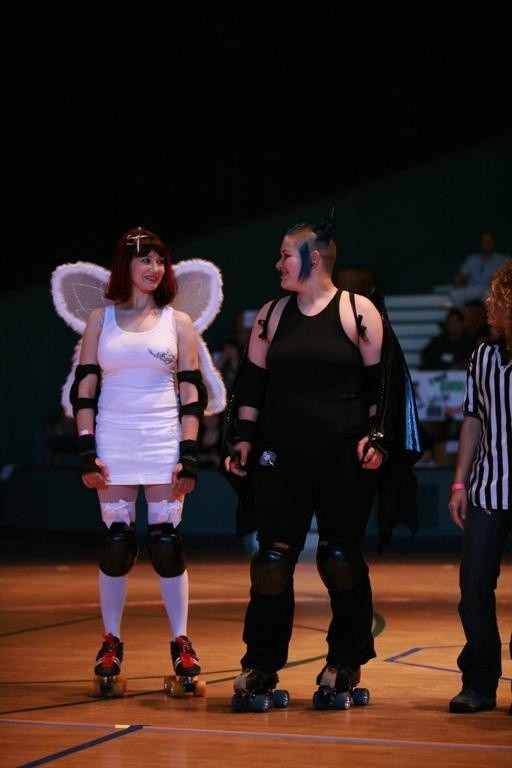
[235,294,455,372]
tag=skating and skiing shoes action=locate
[164,635,206,697]
[87,632,127,698]
[313,662,370,710]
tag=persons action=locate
[223,220,394,693]
[447,258,512,715]
[71,223,207,679]
[69,232,512,471]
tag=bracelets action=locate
[450,482,466,493]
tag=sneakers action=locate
[231,668,290,713]
[450,688,496,713]
